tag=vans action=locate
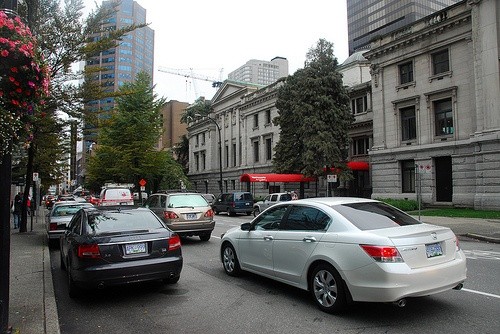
[252,192,293,218]
[98,183,134,206]
[132,193,148,202]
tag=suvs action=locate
[212,192,254,217]
[146,190,216,242]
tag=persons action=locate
[11,195,22,229]
[18,192,31,215]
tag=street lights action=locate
[195,113,224,195]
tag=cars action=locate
[46,203,97,241]
[202,194,216,207]
[57,204,184,297]
[42,192,99,222]
[221,197,467,314]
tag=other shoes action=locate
[14,227,17,229]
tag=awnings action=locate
[240,173,317,200]
[322,161,369,174]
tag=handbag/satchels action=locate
[11,201,14,213]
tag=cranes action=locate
[157,65,222,83]
